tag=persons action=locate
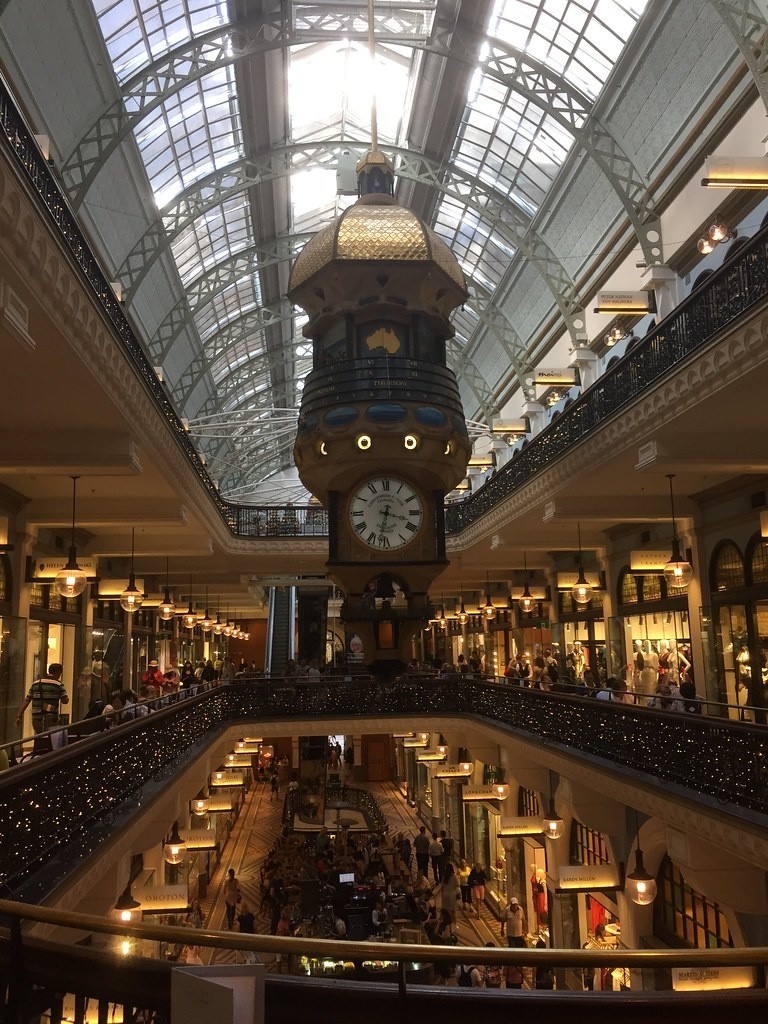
[83,649,767,734]
[178,740,609,991]
[528,862,539,913]
[551,640,693,706]
[535,869,549,928]
[15,663,69,746]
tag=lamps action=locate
[112,882,143,924]
[162,821,187,865]
[482,571,497,620]
[435,734,448,757]
[416,732,430,743]
[492,746,510,800]
[542,769,565,839]
[53,476,87,598]
[662,474,693,587]
[212,766,226,784]
[157,557,251,641]
[626,808,657,905]
[237,738,246,749]
[190,789,211,816]
[519,552,536,613]
[120,527,144,612]
[459,749,474,776]
[572,524,592,602]
[425,582,468,630]
[225,750,239,765]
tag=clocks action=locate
[346,475,422,552]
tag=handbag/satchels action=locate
[456,893,461,900]
[237,894,242,903]
[523,938,531,948]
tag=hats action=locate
[511,898,518,904]
[149,660,158,667]
[166,665,174,673]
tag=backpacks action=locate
[542,970,554,985]
[458,965,474,987]
[485,966,501,985]
[271,776,277,785]
[508,966,522,984]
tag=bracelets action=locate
[17,717,22,718]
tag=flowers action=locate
[142,670,166,689]
[348,881,382,899]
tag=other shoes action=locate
[470,908,473,912]
[271,798,272,801]
[463,906,466,909]
[276,798,278,799]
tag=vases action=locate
[147,684,158,699]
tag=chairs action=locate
[73,701,105,734]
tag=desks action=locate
[242,672,281,684]
[377,838,397,855]
[382,853,411,884]
